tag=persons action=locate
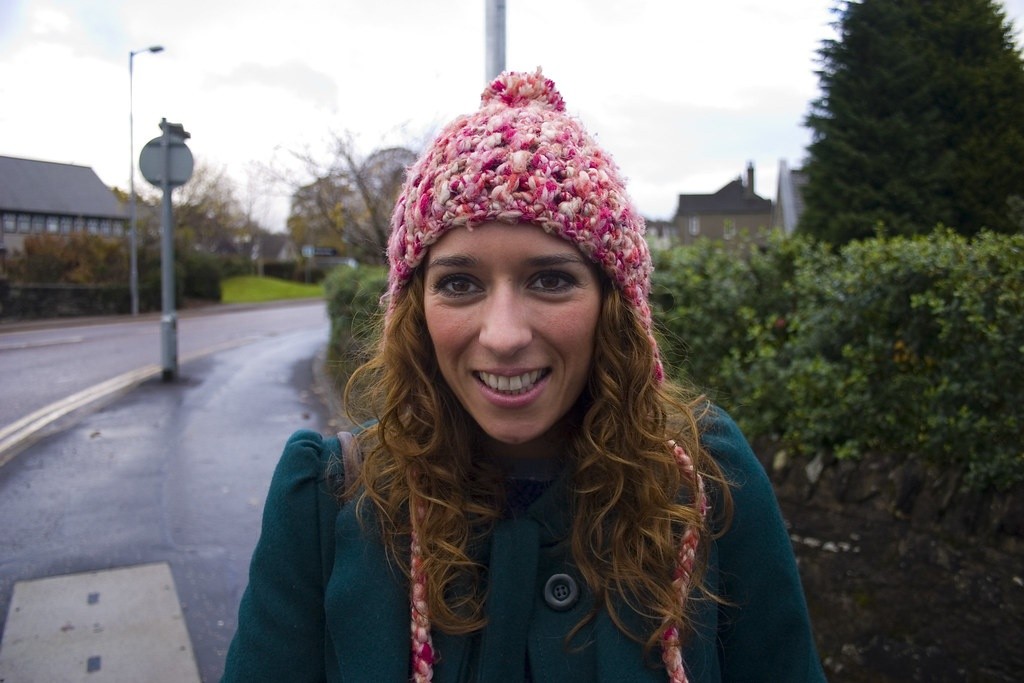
[217,71,833,683]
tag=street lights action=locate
[129,46,166,316]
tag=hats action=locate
[384,63,709,683]
